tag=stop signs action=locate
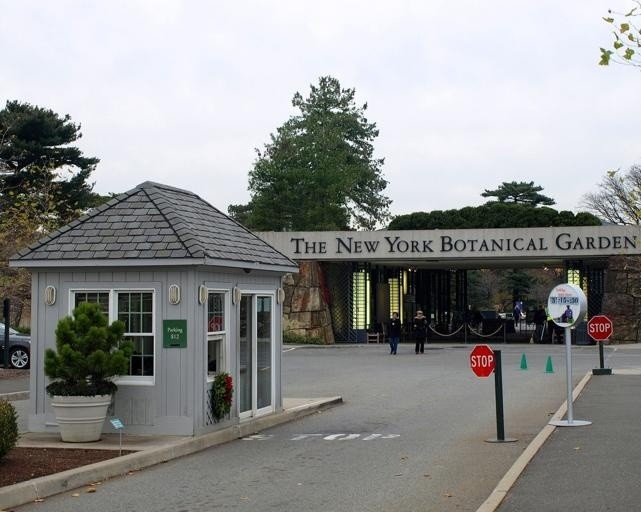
[209,315,223,331]
[587,315,613,342]
[470,343,495,378]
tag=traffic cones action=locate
[521,351,528,369]
[546,354,555,373]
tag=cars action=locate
[0,322,32,369]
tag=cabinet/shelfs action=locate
[366,332,379,344]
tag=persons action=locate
[534,304,548,343]
[389,312,401,355]
[514,304,522,327]
[413,311,431,353]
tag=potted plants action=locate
[43,302,134,444]
